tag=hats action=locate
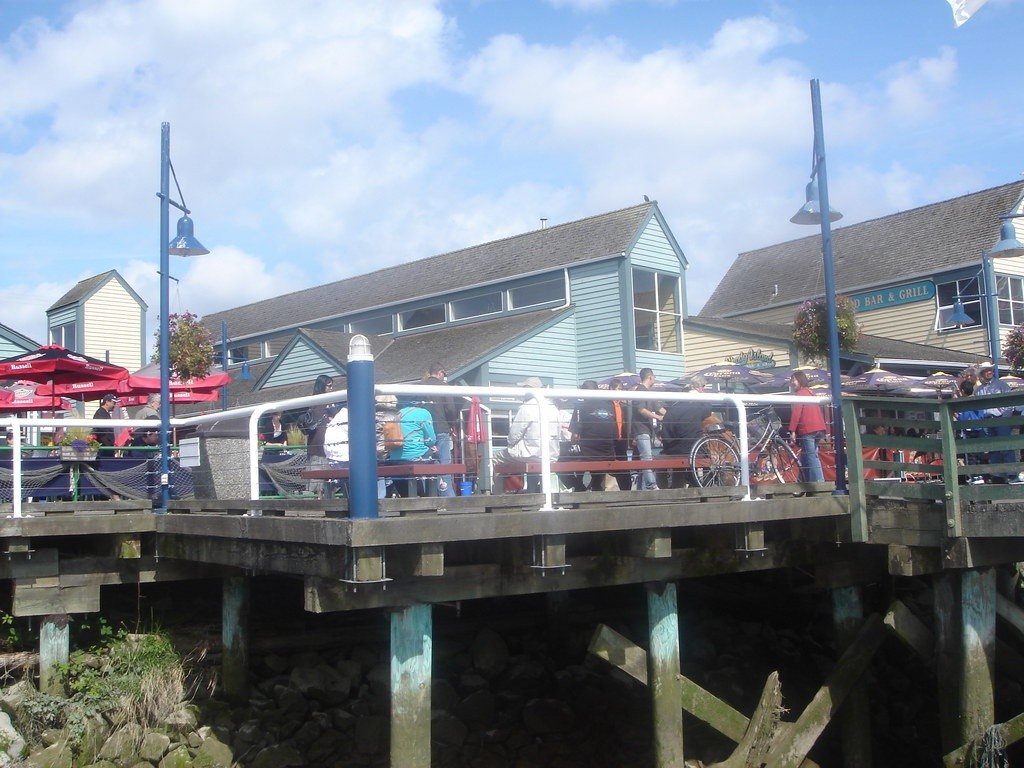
[517,377,543,388]
[103,394,122,403]
[979,362,995,368]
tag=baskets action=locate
[748,411,782,438]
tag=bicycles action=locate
[689,404,808,498]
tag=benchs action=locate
[299,462,467,499]
[495,456,722,489]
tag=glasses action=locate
[326,384,332,387]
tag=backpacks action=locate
[383,407,423,450]
[297,408,326,435]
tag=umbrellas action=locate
[467,396,487,477]
[841,367,919,392]
[999,373,1024,387]
[597,371,683,390]
[782,367,832,388]
[808,387,861,397]
[664,361,797,389]
[0,340,233,447]
[915,372,960,388]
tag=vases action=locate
[59,444,100,462]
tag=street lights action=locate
[153,121,210,511]
[214,319,258,412]
[944,252,1016,483]
[789,78,850,495]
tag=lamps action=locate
[348,333,376,362]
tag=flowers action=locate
[58,427,102,449]
[790,290,861,366]
[151,305,219,382]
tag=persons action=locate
[408,362,458,497]
[789,372,826,483]
[547,368,712,492]
[323,393,402,499]
[262,411,288,455]
[952,361,1024,485]
[389,396,448,498]
[128,429,161,459]
[92,394,117,457]
[305,374,338,499]
[135,392,161,420]
[6,433,14,447]
[861,425,927,437]
[490,376,560,495]
